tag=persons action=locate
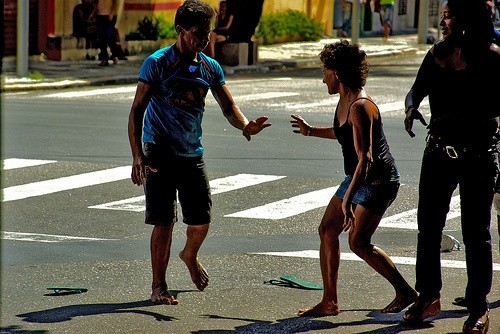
[128,0,272,306]
[333,0,500,334]
[290,40,418,317]
[72,0,233,65]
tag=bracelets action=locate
[307,126,312,136]
[405,105,416,114]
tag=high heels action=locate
[404,294,440,322]
[462,310,490,334]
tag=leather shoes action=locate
[100,60,108,66]
[113,57,118,64]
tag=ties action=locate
[429,141,495,159]
